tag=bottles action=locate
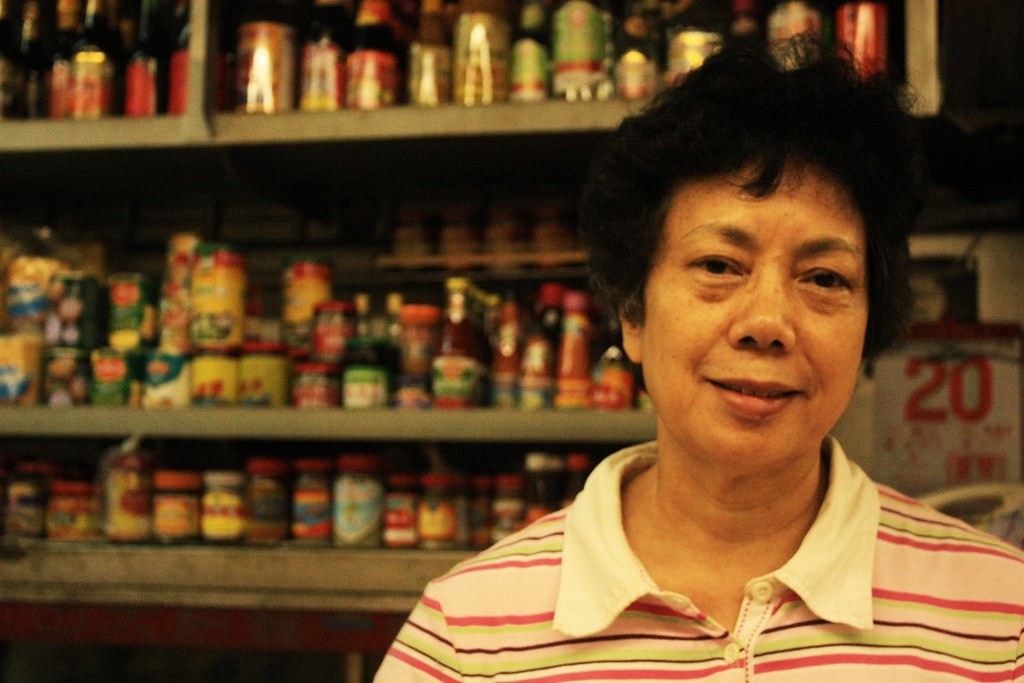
[430,269,634,555]
[1,0,894,123]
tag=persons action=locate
[368,25,1024,683]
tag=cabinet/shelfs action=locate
[0,0,944,615]
